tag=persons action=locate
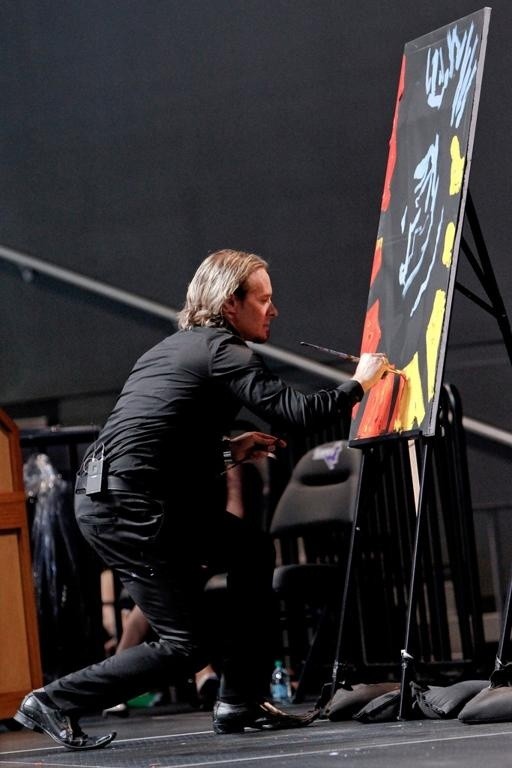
[98,418,266,717]
[12,247,396,752]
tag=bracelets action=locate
[222,437,235,465]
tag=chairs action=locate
[202,438,375,703]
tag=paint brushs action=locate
[219,432,290,477]
[300,341,409,382]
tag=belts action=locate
[74,474,131,493]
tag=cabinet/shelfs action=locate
[0,409,44,721]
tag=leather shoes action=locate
[13,690,118,751]
[211,702,321,734]
